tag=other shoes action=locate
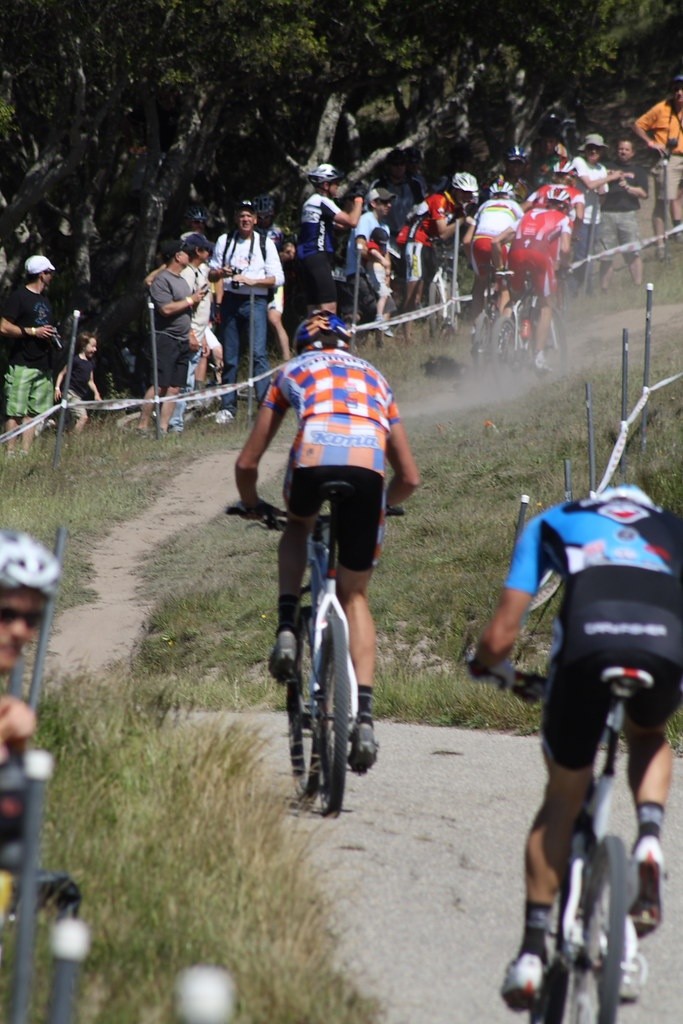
[214,409,234,424]
[379,326,395,338]
[533,357,552,374]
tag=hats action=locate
[671,74,683,84]
[366,187,397,207]
[24,256,55,274]
[181,231,214,253]
[235,200,256,212]
[159,239,180,261]
[577,134,610,152]
[372,228,389,252]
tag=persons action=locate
[1,254,58,460]
[233,308,421,774]
[569,70,683,296]
[136,198,284,436]
[0,527,62,935]
[33,333,102,437]
[274,161,399,348]
[467,484,683,1011]
[365,145,586,375]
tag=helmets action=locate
[386,150,409,163]
[294,310,353,353]
[545,188,571,208]
[552,160,579,177]
[489,181,517,200]
[308,164,346,186]
[185,209,209,221]
[452,172,479,192]
[503,145,530,163]
[0,529,58,600]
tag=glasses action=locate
[0,607,42,628]
[190,219,207,225]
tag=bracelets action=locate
[31,327,36,336]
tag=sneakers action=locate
[501,953,546,1009]
[349,727,376,771]
[629,835,665,938]
[268,631,296,678]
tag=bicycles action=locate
[224,497,408,814]
[460,651,683,1022]
[469,262,569,377]
